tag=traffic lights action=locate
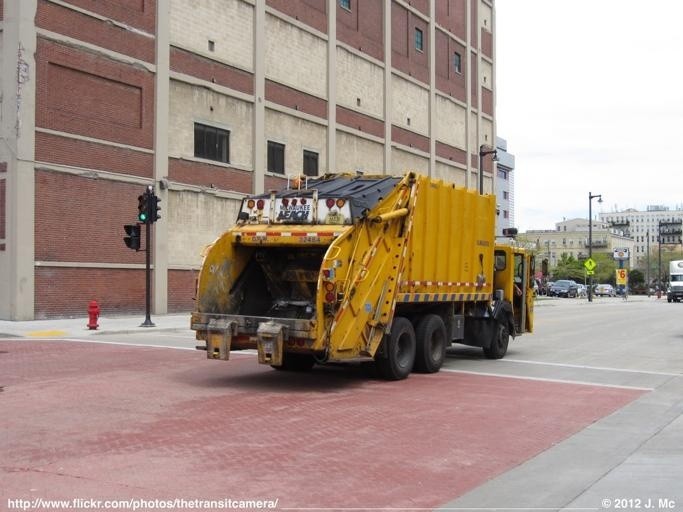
[150,196,161,221]
[136,193,148,222]
[122,224,140,249]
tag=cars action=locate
[529,275,667,302]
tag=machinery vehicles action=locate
[186,171,539,376]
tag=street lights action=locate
[587,192,604,301]
[478,140,497,194]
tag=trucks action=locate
[667,259,683,303]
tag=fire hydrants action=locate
[85,301,100,331]
